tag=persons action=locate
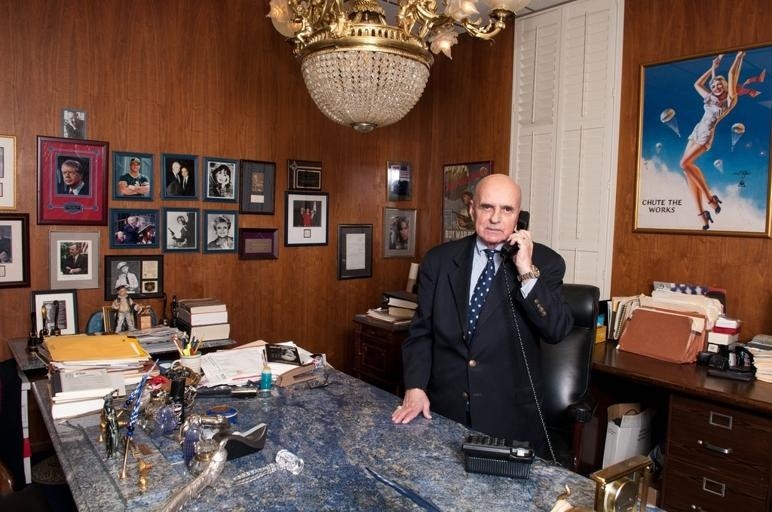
[118,158,150,196]
[300,208,316,228]
[116,216,155,244]
[115,261,139,292]
[391,218,410,251]
[64,244,86,274]
[166,161,194,197]
[112,284,142,333]
[392,173,571,461]
[208,163,231,197]
[59,160,88,195]
[171,215,195,246]
[208,216,233,248]
[681,50,749,230]
[451,190,476,231]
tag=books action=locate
[605,280,772,389]
[175,297,230,342]
[48,370,115,419]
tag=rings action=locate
[397,406,403,409]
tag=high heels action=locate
[698,211,713,230]
[709,196,721,214]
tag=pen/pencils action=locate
[173,331,204,356]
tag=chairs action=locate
[537,280,598,464]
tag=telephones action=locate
[500,210,530,260]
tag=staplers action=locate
[278,362,319,388]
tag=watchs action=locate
[516,266,540,280]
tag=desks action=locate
[6,338,240,483]
[34,349,672,512]
[578,337,771,510]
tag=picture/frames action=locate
[201,208,238,253]
[0,213,31,289]
[102,254,164,301]
[161,205,201,253]
[0,135,17,210]
[386,159,413,201]
[104,306,127,334]
[629,41,771,240]
[284,190,329,247]
[36,136,109,226]
[30,288,79,336]
[202,155,239,203]
[48,229,100,290]
[112,150,154,202]
[440,160,492,245]
[336,222,374,281]
[239,158,276,215]
[108,208,160,249]
[159,152,198,199]
[238,227,278,260]
[381,205,419,258]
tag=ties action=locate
[467,249,500,345]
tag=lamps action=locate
[268,0,533,133]
